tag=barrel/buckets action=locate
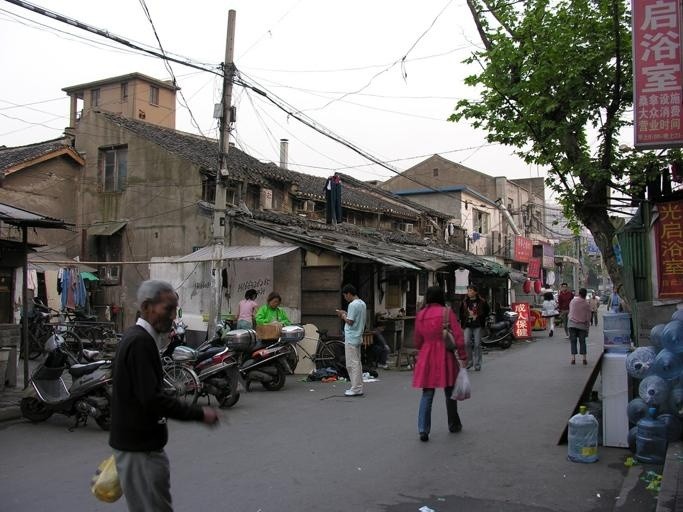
[626,347,656,379]
[660,320,683,354]
[638,376,670,405]
[650,323,665,350]
[672,309,683,322]
[656,412,683,442]
[654,350,681,381]
[627,398,648,424]
[566,406,599,463]
[634,407,668,465]
[583,390,603,445]
[627,425,638,454]
[601,313,632,353]
[668,378,683,422]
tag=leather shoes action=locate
[420,434,428,442]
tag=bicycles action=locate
[284,322,348,376]
[19,298,119,364]
[159,349,201,408]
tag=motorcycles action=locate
[480,311,519,348]
[216,320,305,390]
[160,308,256,409]
[18,325,115,431]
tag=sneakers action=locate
[344,388,364,396]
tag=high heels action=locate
[583,359,588,365]
[571,359,575,365]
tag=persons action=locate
[540,292,560,338]
[589,292,601,327]
[542,282,554,292]
[234,288,258,329]
[606,285,621,313]
[557,282,574,339]
[411,285,471,444]
[254,290,294,326]
[334,283,368,397]
[460,284,490,372]
[566,287,592,366]
[106,278,233,512]
